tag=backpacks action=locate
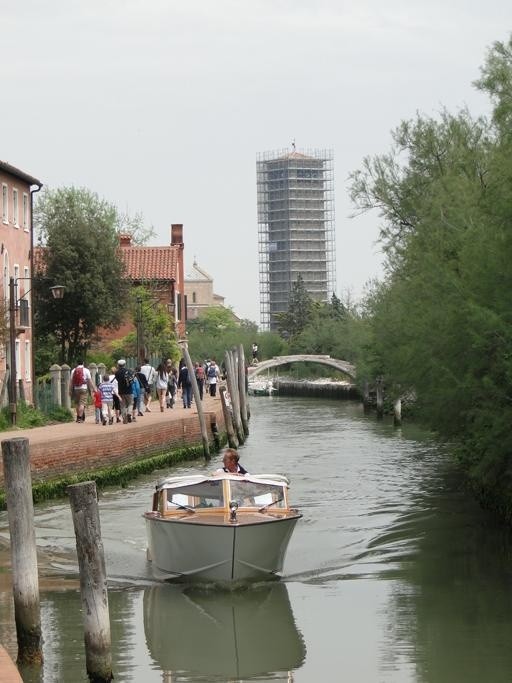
[73,367,84,386]
[208,366,216,377]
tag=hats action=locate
[118,359,126,366]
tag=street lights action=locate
[6,273,69,425]
[133,294,175,370]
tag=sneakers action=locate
[76,419,83,423]
[160,403,173,412]
[184,401,192,408]
[96,414,136,425]
[138,406,151,416]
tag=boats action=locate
[248,367,279,397]
[139,469,306,593]
[138,579,307,682]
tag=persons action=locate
[211,447,256,507]
[250,341,259,366]
[67,356,221,425]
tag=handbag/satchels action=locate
[144,383,150,394]
[198,367,203,378]
[168,374,174,388]
[125,370,134,387]
[186,374,191,385]
